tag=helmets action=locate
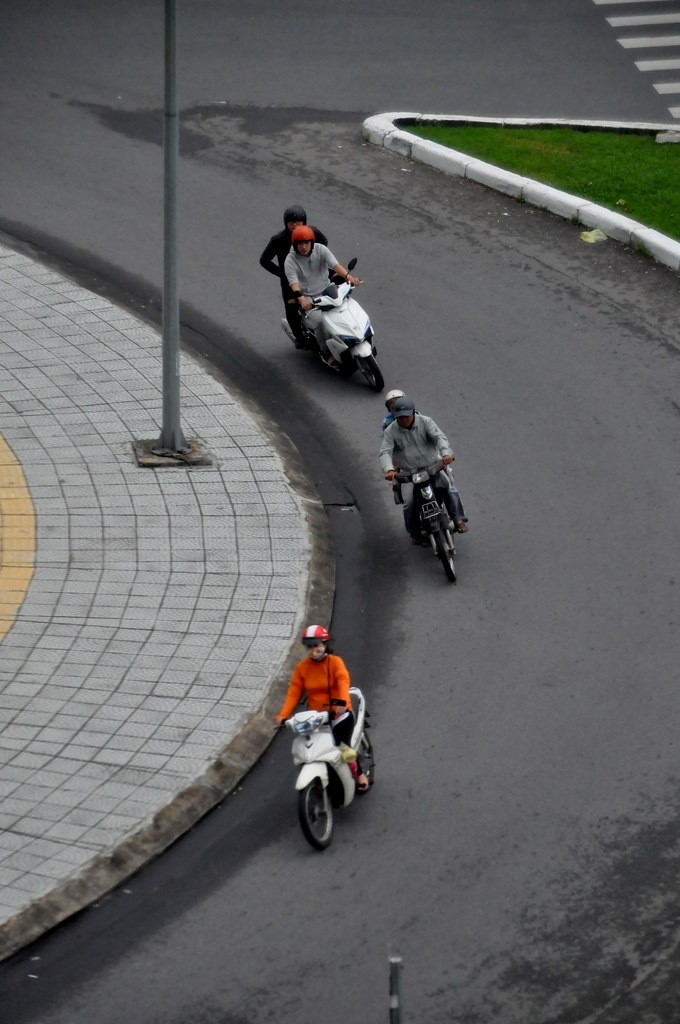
[292,226,314,243]
[302,625,327,642]
[283,205,307,228]
[394,398,414,418]
[385,390,406,409]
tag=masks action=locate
[306,647,324,658]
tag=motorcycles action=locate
[274,686,378,853]
[280,257,385,394]
[383,454,470,583]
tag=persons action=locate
[284,225,362,371]
[379,397,469,546]
[272,625,369,790]
[260,206,355,349]
[381,389,423,440]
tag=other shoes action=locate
[295,335,310,349]
[359,774,368,790]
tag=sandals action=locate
[454,519,468,533]
[413,535,422,544]
